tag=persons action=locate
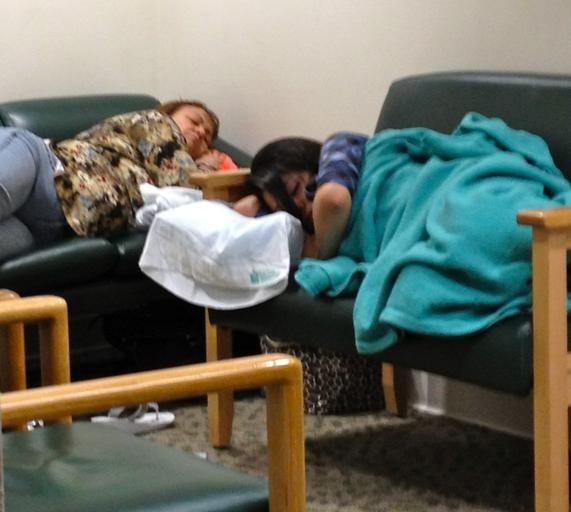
[233,110,570,338]
[0,98,223,262]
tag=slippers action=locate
[91,401,175,437]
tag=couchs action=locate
[190,68,571,512]
[1,92,272,403]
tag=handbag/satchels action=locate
[257,332,384,414]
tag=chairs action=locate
[0,292,306,512]
